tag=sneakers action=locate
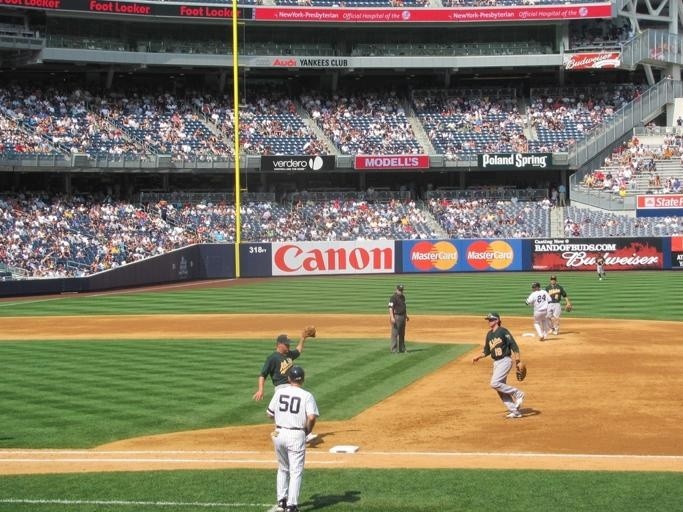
[506,393,525,419]
[540,327,559,341]
[305,432,318,444]
[273,499,301,512]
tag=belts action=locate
[276,425,301,431]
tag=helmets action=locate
[288,366,305,383]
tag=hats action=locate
[397,285,405,290]
[485,312,500,321]
[550,275,556,280]
[532,282,540,288]
[278,335,293,345]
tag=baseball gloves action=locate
[303,326,316,338]
[516,360,526,381]
[565,303,572,311]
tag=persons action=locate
[1,72,649,161]
[470,313,527,418]
[1,181,568,283]
[566,216,682,236]
[263,364,321,512]
[1,1,683,61]
[387,284,409,352]
[581,116,683,196]
[524,279,552,341]
[543,274,571,336]
[251,329,318,442]
[595,252,607,281]
